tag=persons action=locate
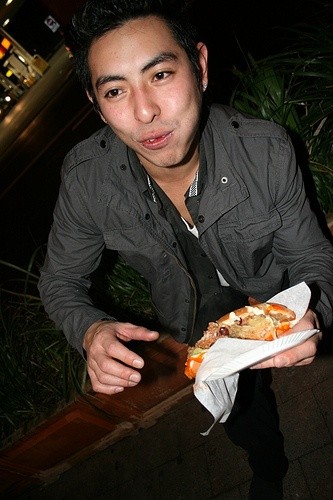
[36,2,333,500]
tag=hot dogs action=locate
[183,302,296,379]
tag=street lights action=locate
[0,17,33,61]
